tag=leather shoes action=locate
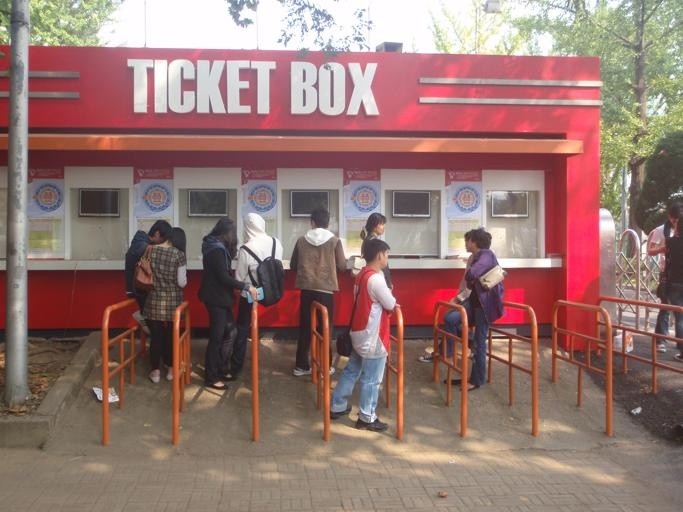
[149,374,160,383]
[166,373,173,382]
[443,378,463,386]
[204,379,228,390]
[221,373,237,380]
[461,383,481,393]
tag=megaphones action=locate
[376,42,403,53]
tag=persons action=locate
[646,202,683,353]
[417,229,473,363]
[289,208,346,376]
[197,216,258,390]
[330,239,400,432]
[665,217,683,362]
[125,220,170,369]
[227,213,283,375]
[447,227,505,391]
[360,213,394,291]
[143,227,187,384]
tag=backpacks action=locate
[239,237,284,306]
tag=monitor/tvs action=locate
[392,190,431,217]
[291,190,330,217]
[79,189,120,218]
[492,191,528,218]
[188,189,229,217]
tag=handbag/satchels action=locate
[132,244,154,295]
[473,250,509,292]
[335,329,353,356]
[350,237,375,275]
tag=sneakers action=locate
[417,351,436,364]
[330,403,351,420]
[657,340,683,363]
[292,367,312,377]
[321,366,335,378]
[356,417,389,432]
[132,311,151,336]
